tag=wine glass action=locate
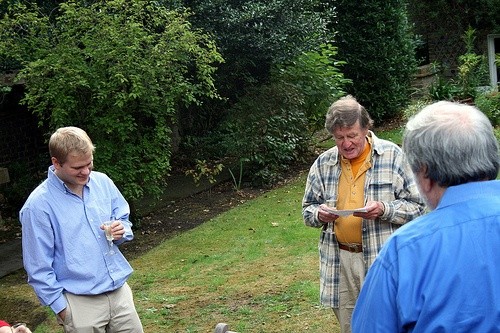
[322,194,336,234]
[103,216,118,256]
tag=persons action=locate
[19,126,144,333]
[302,94,426,333]
[0,320,32,333]
[351,100,500,333]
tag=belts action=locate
[339,243,362,253]
